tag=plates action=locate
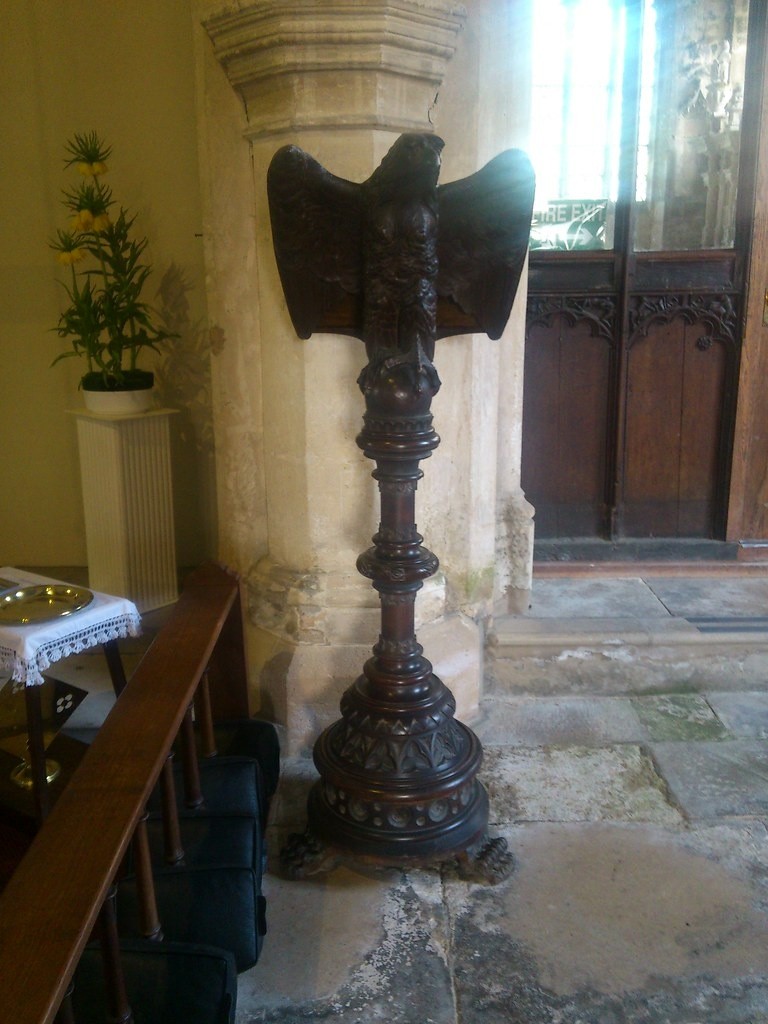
[0,585,93,624]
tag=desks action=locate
[0,567,143,825]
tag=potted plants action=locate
[44,128,181,415]
